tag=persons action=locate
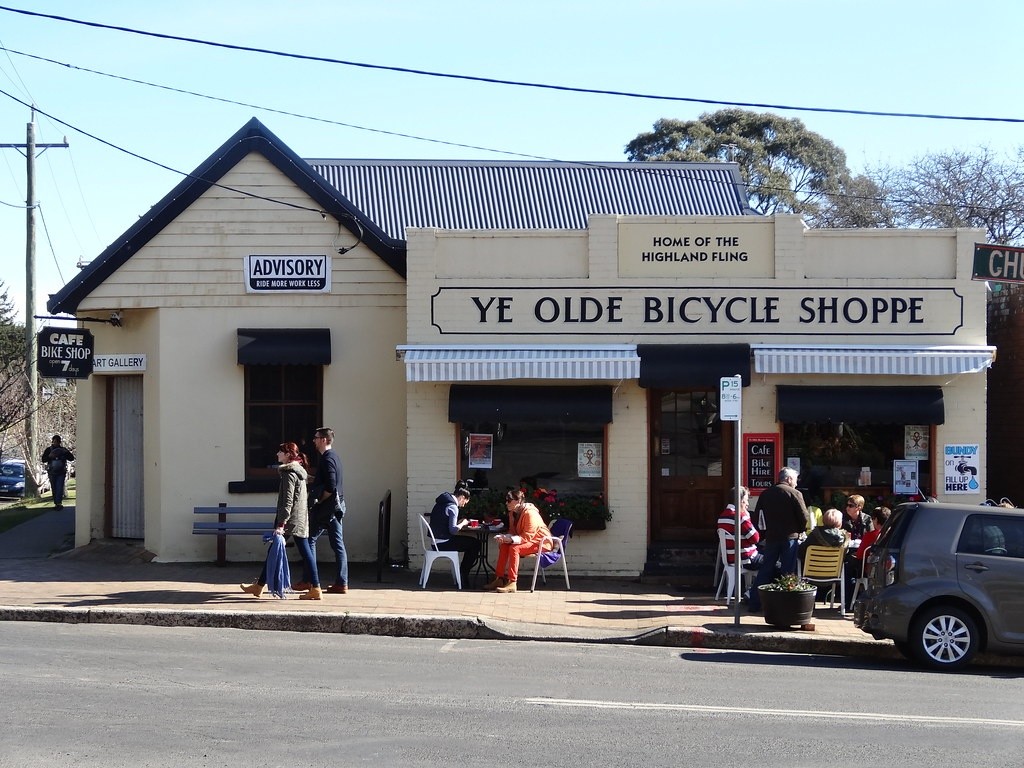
[798,494,892,612]
[972,502,1024,557]
[718,486,781,604]
[428,488,481,587]
[958,456,967,465]
[291,428,348,594]
[748,468,809,616]
[484,490,553,592]
[42,435,74,510]
[241,443,322,599]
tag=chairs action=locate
[850,545,871,610]
[520,520,574,592]
[796,545,846,616]
[714,528,759,605]
[713,534,722,588]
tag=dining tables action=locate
[759,536,862,593]
[459,522,503,580]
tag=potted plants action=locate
[758,571,818,624]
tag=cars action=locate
[0,433,76,500]
[853,502,1024,671]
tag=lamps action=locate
[497,420,507,441]
[464,435,469,459]
[418,512,462,589]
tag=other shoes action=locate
[323,584,345,594]
[496,580,517,593]
[292,581,317,590]
[299,588,323,600]
[56,504,61,511]
[456,577,471,590]
[484,577,504,589]
[744,590,750,600]
[241,584,263,596]
[837,606,841,613]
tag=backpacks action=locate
[50,446,65,473]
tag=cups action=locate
[470,519,479,527]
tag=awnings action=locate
[237,328,331,365]
[754,348,993,375]
[775,385,945,425]
[638,343,751,388]
[450,384,614,423]
[404,350,641,381]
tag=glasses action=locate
[314,436,320,440]
[847,503,855,508]
[507,498,513,503]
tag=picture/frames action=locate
[893,459,918,494]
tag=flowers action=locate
[459,481,602,531]
[876,488,931,511]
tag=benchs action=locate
[193,503,329,567]
